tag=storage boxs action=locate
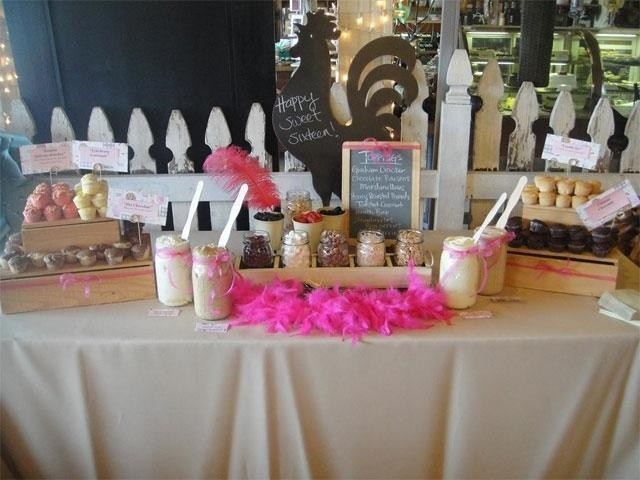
[522,204,603,229]
[0,234,157,314]
[505,247,619,297]
[21,218,121,252]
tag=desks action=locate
[0,231,639,480]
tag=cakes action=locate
[1,221,151,275]
[521,173,603,211]
[23,174,108,223]
[505,209,634,258]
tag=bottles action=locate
[355,230,387,266]
[317,230,350,266]
[154,235,193,307]
[395,228,425,267]
[278,230,312,268]
[438,225,508,309]
[192,245,232,321]
[285,189,312,233]
[242,230,273,267]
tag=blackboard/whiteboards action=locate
[340,141,420,247]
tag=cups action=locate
[252,206,347,250]
